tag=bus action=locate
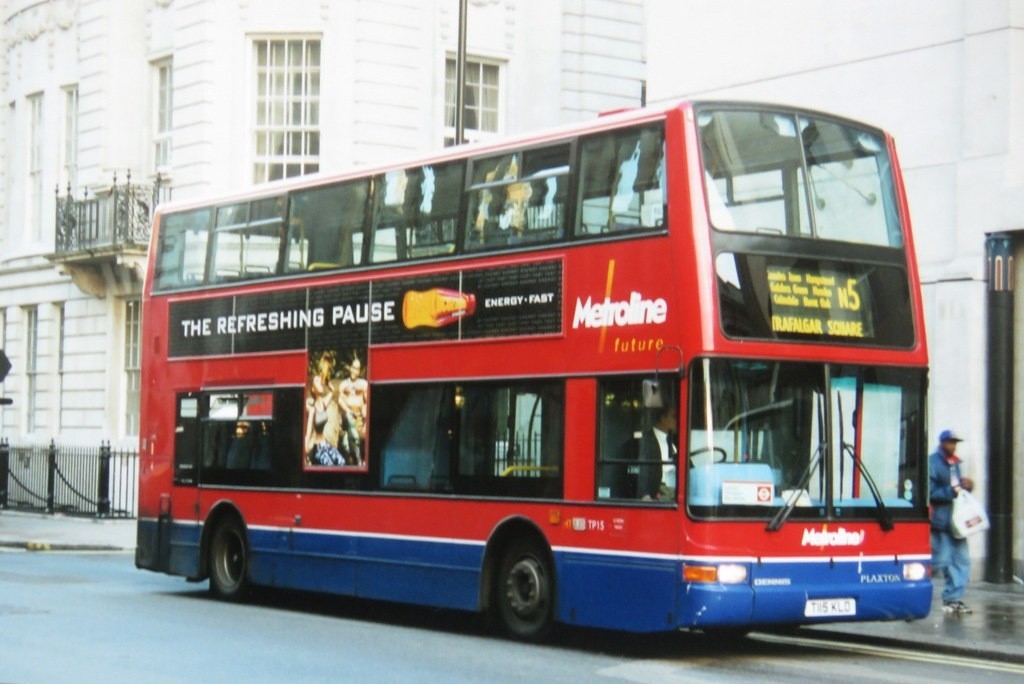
[135,95,932,645]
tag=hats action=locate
[940,430,963,443]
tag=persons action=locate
[927,430,975,614]
[635,405,695,501]
[304,349,368,468]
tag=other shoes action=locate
[942,600,971,613]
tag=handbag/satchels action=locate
[950,488,991,539]
